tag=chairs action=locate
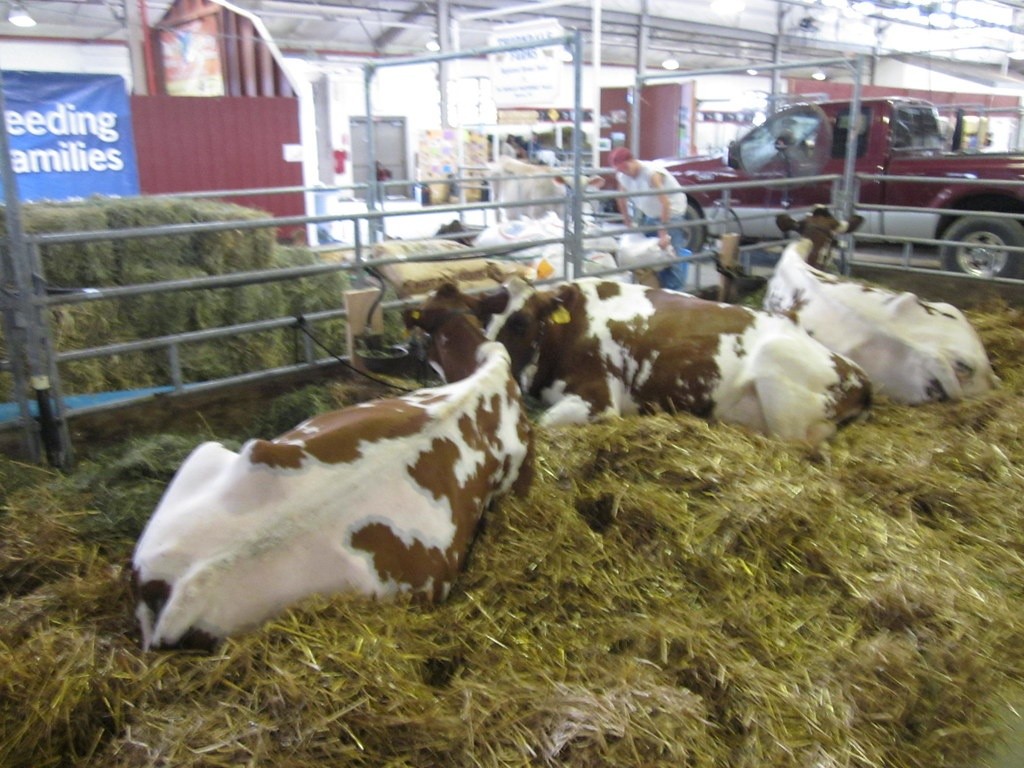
[830,125,848,159]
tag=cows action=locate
[485,268,873,447]
[487,155,607,220]
[128,275,530,635]
[765,203,1006,406]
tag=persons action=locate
[503,135,527,159]
[611,147,689,290]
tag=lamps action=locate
[7,1,38,28]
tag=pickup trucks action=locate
[584,97,1024,282]
